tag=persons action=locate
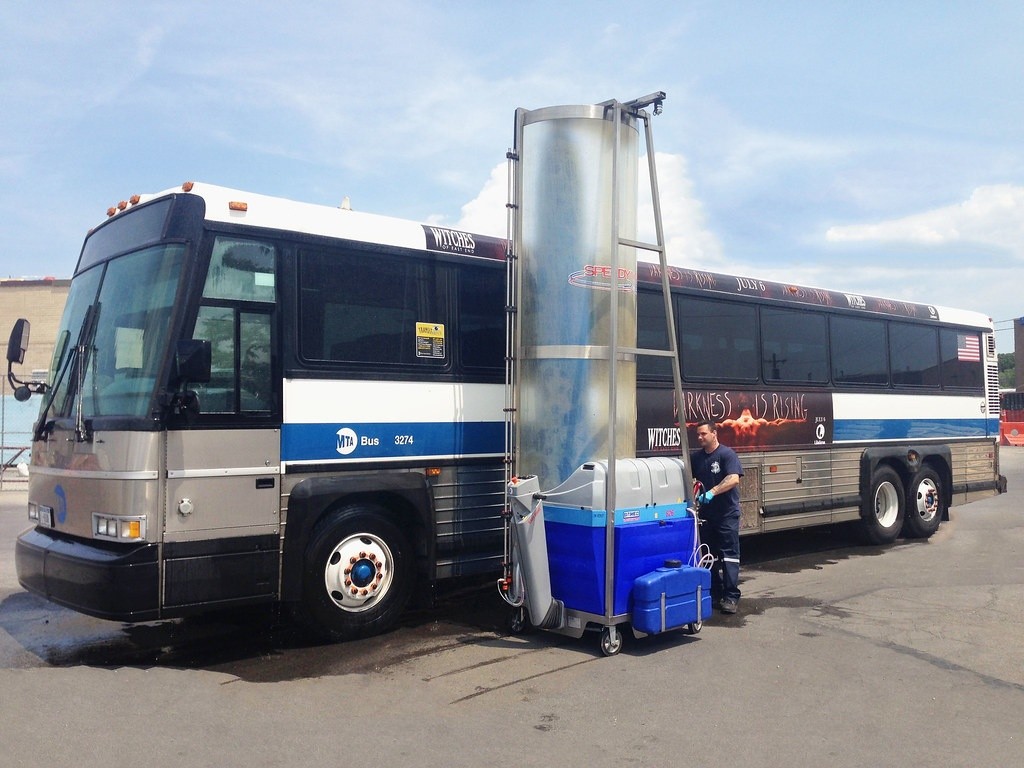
[690,420,745,613]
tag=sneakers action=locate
[712,598,720,608]
[720,598,738,614]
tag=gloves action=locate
[699,490,714,504]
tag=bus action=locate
[7,180,1007,643]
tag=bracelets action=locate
[713,487,719,494]
